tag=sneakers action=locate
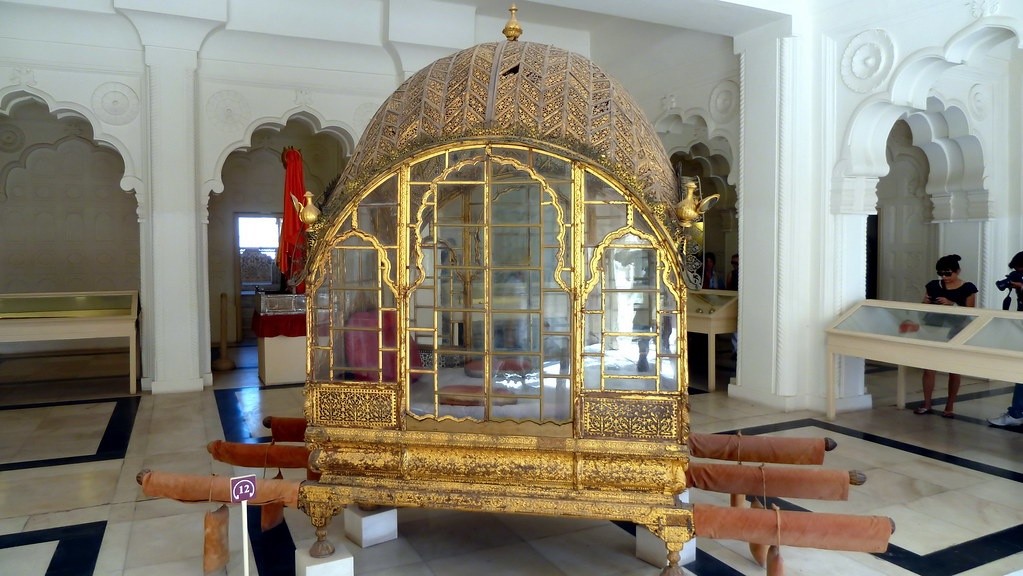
[987,413,1023,426]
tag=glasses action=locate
[937,271,953,277]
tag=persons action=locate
[616,248,672,373]
[727,254,738,290]
[704,252,725,289]
[912,254,979,419]
[987,251,1023,427]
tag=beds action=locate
[339,304,679,440]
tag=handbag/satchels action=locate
[923,312,945,327]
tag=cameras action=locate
[995,271,1023,292]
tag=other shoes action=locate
[637,356,649,372]
[943,410,954,418]
[914,407,928,414]
